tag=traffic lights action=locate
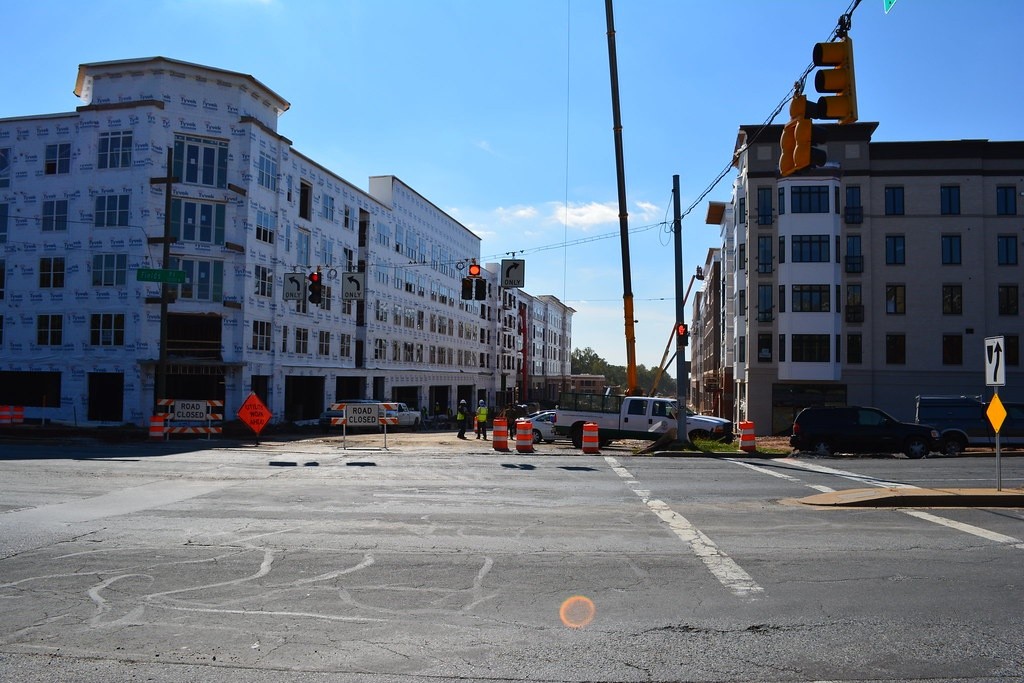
[308,272,322,304]
[675,322,688,346]
[468,264,481,276]
[791,99,827,177]
[813,36,859,127]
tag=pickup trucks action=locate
[386,402,422,433]
[551,391,734,449]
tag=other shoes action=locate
[483,437,486,440]
[457,434,466,440]
[476,437,480,439]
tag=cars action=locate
[526,410,573,445]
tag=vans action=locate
[319,399,381,436]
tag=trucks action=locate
[913,395,1024,458]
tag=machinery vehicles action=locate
[607,0,706,397]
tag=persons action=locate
[434,402,440,418]
[423,406,428,420]
[504,402,516,440]
[476,400,488,440]
[447,405,453,420]
[456,399,469,439]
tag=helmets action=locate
[460,400,466,404]
[479,400,484,406]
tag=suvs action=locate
[790,404,945,460]
